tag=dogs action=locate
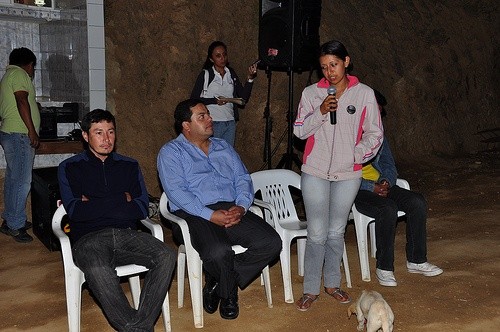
[346,287,395,332]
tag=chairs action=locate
[52,201,172,332]
[160,192,273,328]
[248,170,353,303]
[350,178,411,281]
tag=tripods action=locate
[275,71,303,205]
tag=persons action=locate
[0,48,40,242]
[191,42,257,149]
[293,40,383,311]
[58,109,175,332]
[157,100,282,319]
[354,90,443,287]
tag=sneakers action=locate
[406,261,444,276]
[374,268,398,287]
[6,226,34,243]
[0,220,33,235]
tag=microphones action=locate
[327,86,336,125]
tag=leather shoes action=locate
[218,280,239,320]
[203,271,221,314]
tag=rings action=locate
[254,70,256,72]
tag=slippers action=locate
[296,294,320,311]
[324,287,351,303]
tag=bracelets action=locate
[247,78,251,80]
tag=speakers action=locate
[258,0,321,74]
[32,166,64,251]
